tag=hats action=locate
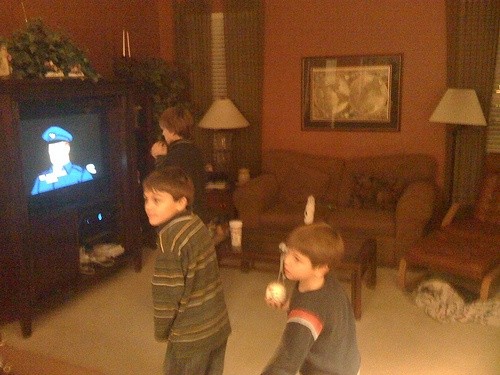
[42,126,72,142]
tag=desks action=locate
[213,228,377,322]
[197,174,239,263]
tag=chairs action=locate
[398,152,500,304]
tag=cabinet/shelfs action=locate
[0,74,187,339]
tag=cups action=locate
[237,169,250,184]
[229,220,243,247]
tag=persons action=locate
[31,126,93,195]
[260,223,362,375]
[143,166,232,375]
[151,105,206,217]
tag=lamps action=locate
[428,88,487,225]
[197,99,249,181]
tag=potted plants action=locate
[0,18,98,82]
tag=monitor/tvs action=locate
[18,107,110,217]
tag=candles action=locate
[122,30,131,58]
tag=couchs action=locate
[234,149,437,270]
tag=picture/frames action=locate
[301,53,400,132]
